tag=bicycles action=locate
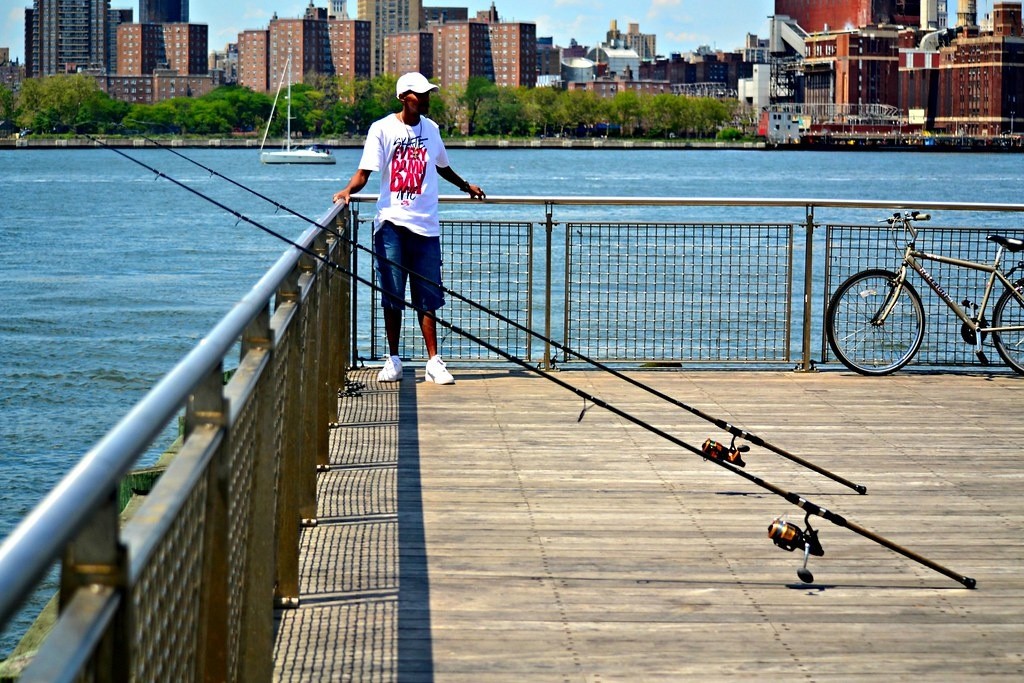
[826,211,1024,377]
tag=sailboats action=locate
[260,53,335,164]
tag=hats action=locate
[396,72,440,98]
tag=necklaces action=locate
[400,111,422,158]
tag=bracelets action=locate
[460,181,469,191]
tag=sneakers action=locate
[377,355,403,381]
[425,354,454,384]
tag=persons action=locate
[332,72,486,384]
[596,59,634,80]
[835,138,1020,147]
[311,146,330,154]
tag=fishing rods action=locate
[128,121,869,499]
[13,98,988,594]
